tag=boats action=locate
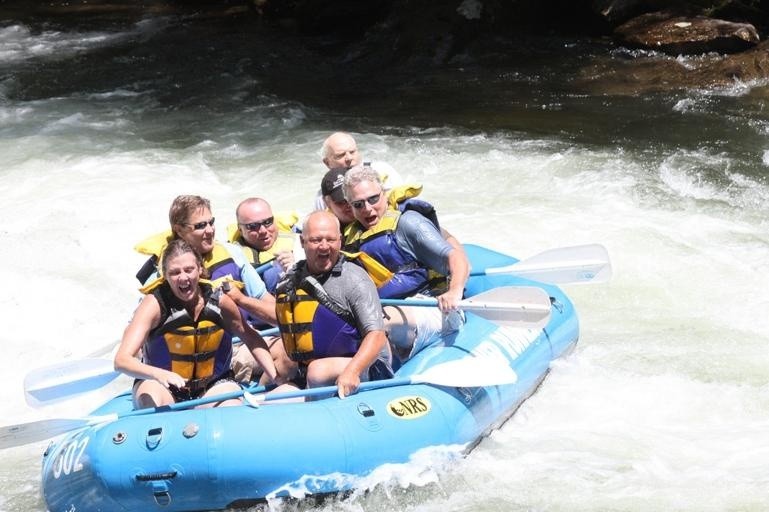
[41,244,578,512]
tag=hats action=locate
[321,167,353,204]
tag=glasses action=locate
[350,190,382,210]
[184,217,216,230]
[239,216,274,231]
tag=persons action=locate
[113,239,288,410]
[213,195,308,303]
[131,193,301,388]
[218,210,394,403]
[335,164,471,380]
[320,165,467,257]
[310,132,397,211]
[493,346,496,351]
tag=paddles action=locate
[31,257,278,402]
[380,285,552,322]
[0,385,277,451]
[244,349,516,408]
